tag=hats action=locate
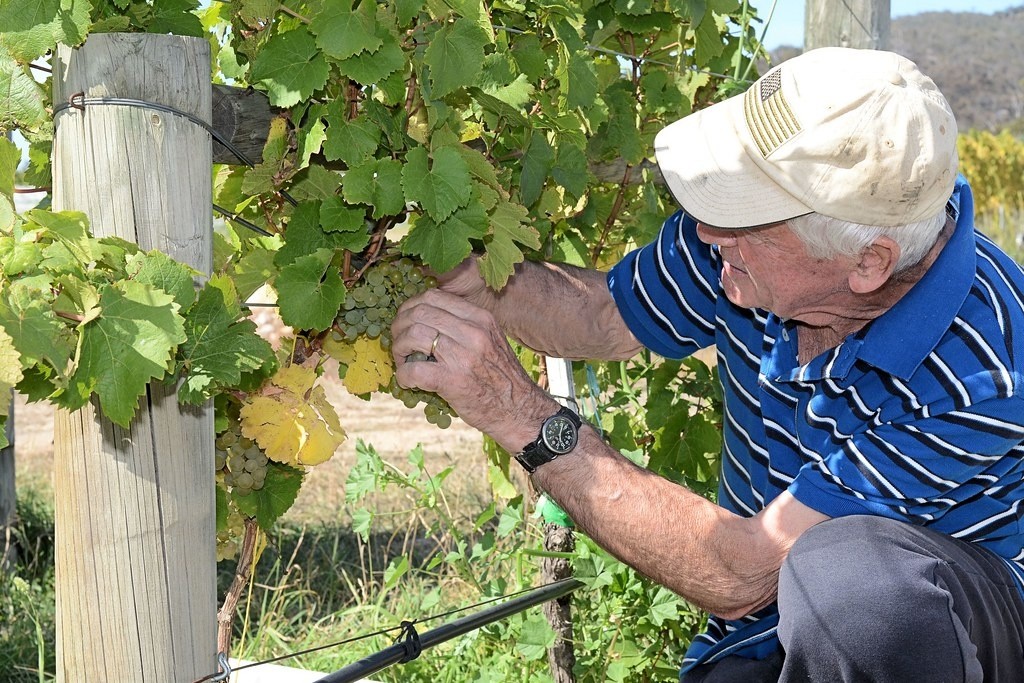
[653,47,959,229]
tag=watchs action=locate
[514,406,582,473]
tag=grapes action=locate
[214,404,267,496]
[335,258,460,429]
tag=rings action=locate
[431,332,442,355]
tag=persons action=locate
[390,46,1023,683]
